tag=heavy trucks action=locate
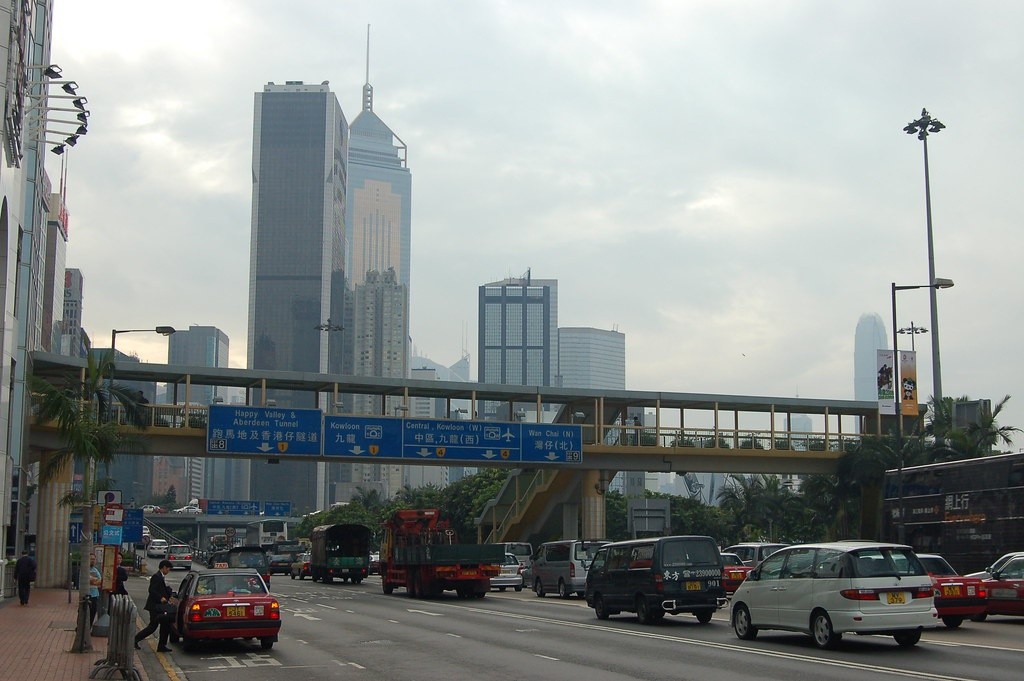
[378,507,506,600]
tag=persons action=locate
[138,391,149,404]
[75,554,102,634]
[107,553,129,615]
[14,550,37,606]
[634,417,641,446]
[134,560,177,653]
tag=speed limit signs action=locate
[225,526,236,537]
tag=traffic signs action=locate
[401,417,523,463]
[205,404,323,458]
[323,415,402,459]
[521,421,583,463]
[207,498,260,516]
[264,500,292,517]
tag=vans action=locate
[492,541,533,570]
[528,539,617,599]
[202,545,271,592]
[581,535,727,627]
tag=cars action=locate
[173,506,203,515]
[290,552,312,580]
[720,552,755,592]
[729,541,939,652]
[969,554,1024,623]
[163,545,192,570]
[147,539,169,558]
[368,555,380,573]
[860,553,989,629]
[722,542,790,567]
[133,525,151,549]
[964,552,1024,581]
[171,568,281,654]
[139,504,169,514]
[489,553,524,592]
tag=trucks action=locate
[258,540,309,576]
[310,523,373,586]
[188,498,209,514]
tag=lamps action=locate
[30,117,88,136]
[333,401,344,408]
[398,404,408,410]
[29,106,91,124]
[27,81,79,95]
[265,399,276,406]
[27,65,63,78]
[26,94,88,114]
[454,408,468,419]
[213,396,223,402]
[28,139,66,155]
[29,128,80,146]
[516,410,525,418]
[570,412,585,423]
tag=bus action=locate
[879,451,1024,577]
[245,519,287,547]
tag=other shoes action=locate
[20,598,28,605]
[133,640,142,650]
[157,647,172,653]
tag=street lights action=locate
[392,405,408,417]
[455,408,469,421]
[314,318,345,414]
[897,321,929,353]
[902,108,947,464]
[570,412,586,424]
[892,277,955,546]
[91,325,177,638]
[513,411,526,422]
